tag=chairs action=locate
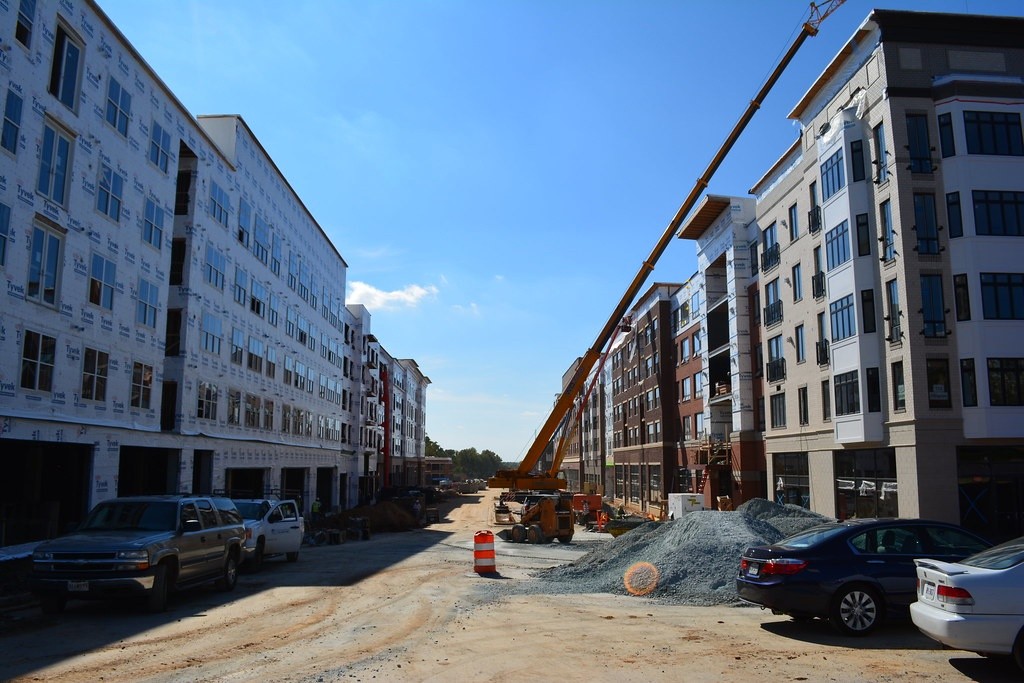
[876,530,897,553]
[901,534,914,552]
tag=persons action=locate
[883,530,902,551]
[312,498,321,518]
[413,500,421,519]
[259,501,282,523]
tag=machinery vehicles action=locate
[496,495,575,544]
[487,0,850,504]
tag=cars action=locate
[907,535,1023,672]
[734,518,996,637]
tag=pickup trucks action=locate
[230,497,304,569]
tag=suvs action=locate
[31,493,247,613]
